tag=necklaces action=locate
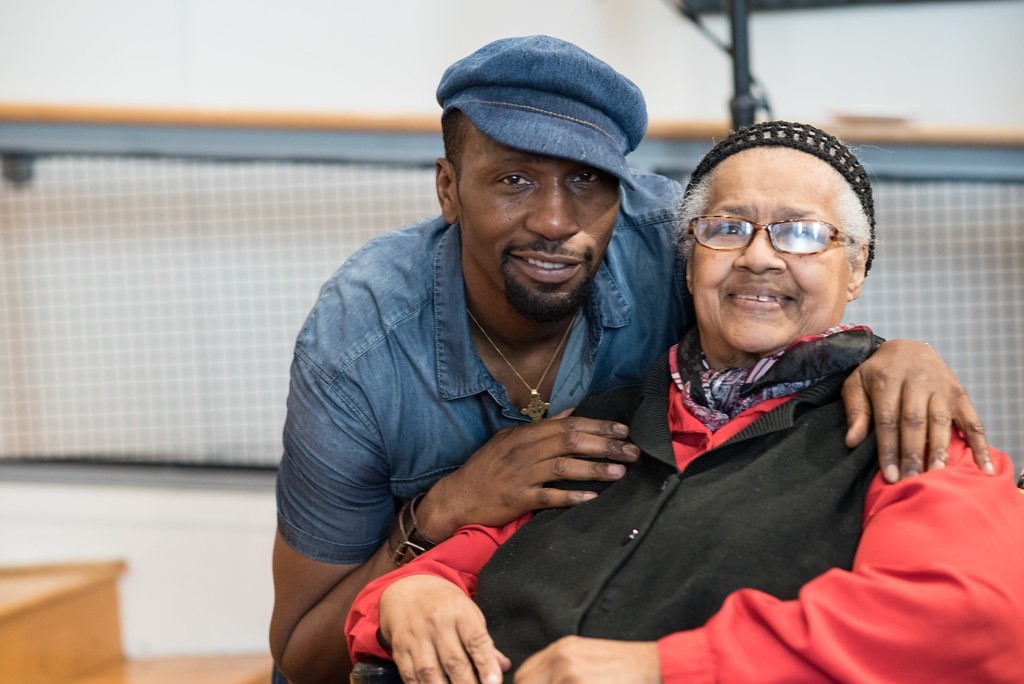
[467,309,576,421]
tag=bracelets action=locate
[389,493,439,566]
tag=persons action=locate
[268,36,994,684]
[342,120,1024,684]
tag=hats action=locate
[437,37,649,193]
[682,119,875,279]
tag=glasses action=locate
[689,214,860,256]
